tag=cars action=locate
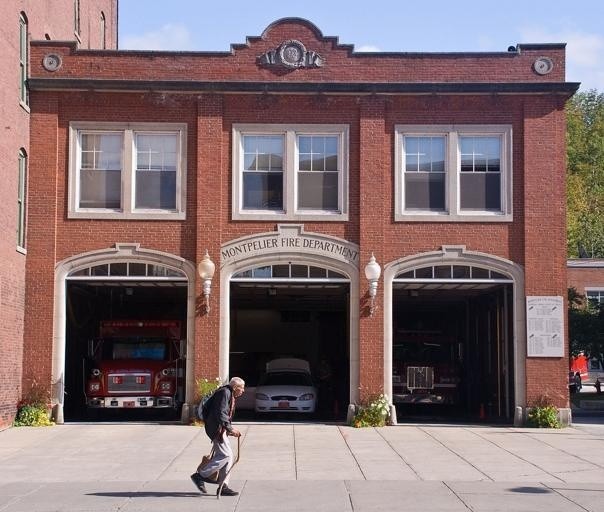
[234,356,320,416]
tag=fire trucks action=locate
[80,315,187,417]
[393,327,469,410]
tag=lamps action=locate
[364,252,382,316]
[197,248,216,314]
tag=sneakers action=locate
[217,487,238,496]
[191,473,208,493]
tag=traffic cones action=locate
[479,403,488,420]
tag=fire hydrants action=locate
[594,379,601,394]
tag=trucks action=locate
[569,350,588,393]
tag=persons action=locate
[575,372,582,393]
[190,377,246,496]
[568,370,577,394]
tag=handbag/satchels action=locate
[197,455,219,482]
